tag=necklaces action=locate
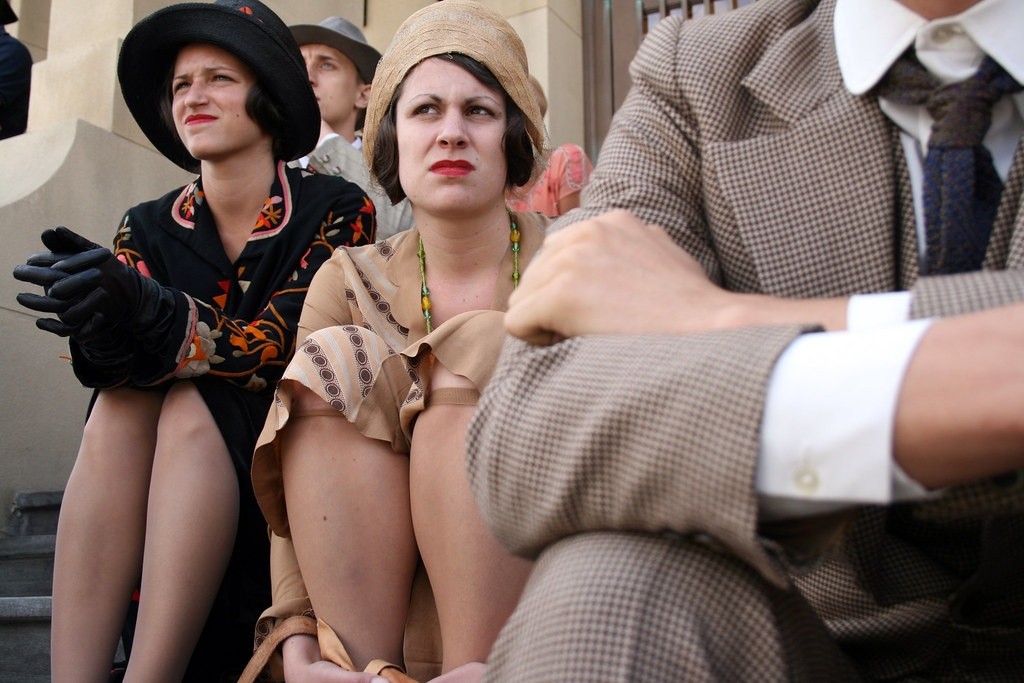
[418,208,520,336]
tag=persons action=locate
[284,16,413,243]
[0,0,33,141]
[465,0,1024,683]
[13,0,377,682]
[251,0,555,683]
[505,75,594,220]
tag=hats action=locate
[287,17,382,84]
[116,0,320,174]
[363,0,544,177]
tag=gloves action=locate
[11,226,189,390]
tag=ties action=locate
[877,45,1023,274]
